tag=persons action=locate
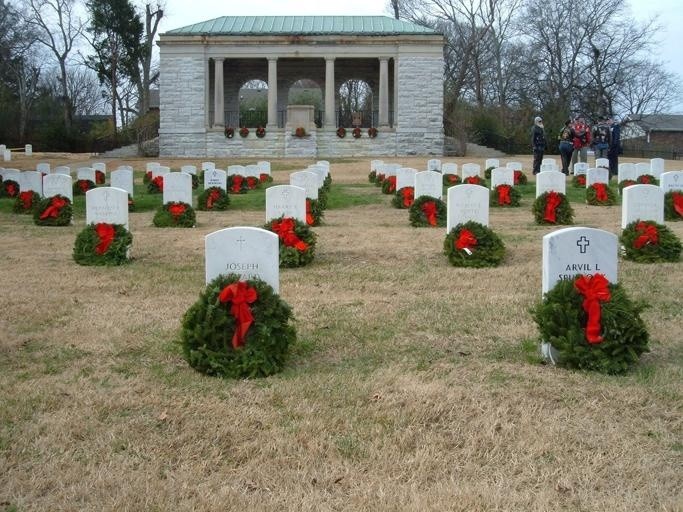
[530,116,547,176]
[604,117,623,176]
[557,119,585,177]
[590,115,612,180]
[569,117,591,174]
